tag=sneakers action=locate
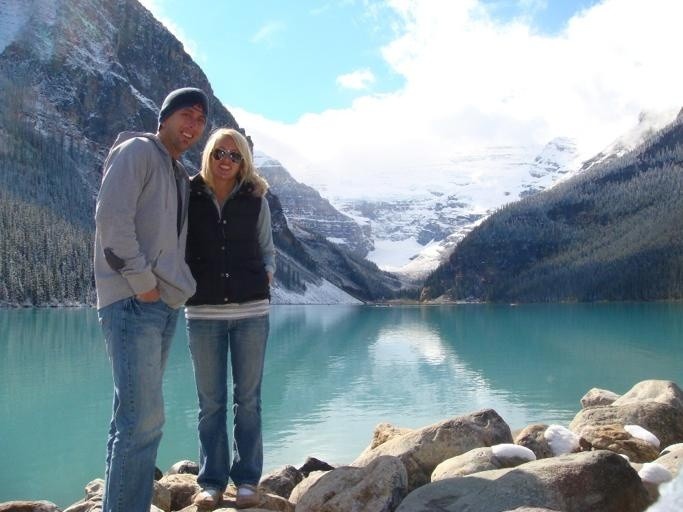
[195,486,224,507]
[236,483,260,509]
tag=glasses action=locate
[212,149,242,164]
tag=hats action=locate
[158,88,210,129]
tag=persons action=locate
[93,87,208,511]
[184,128,277,508]
